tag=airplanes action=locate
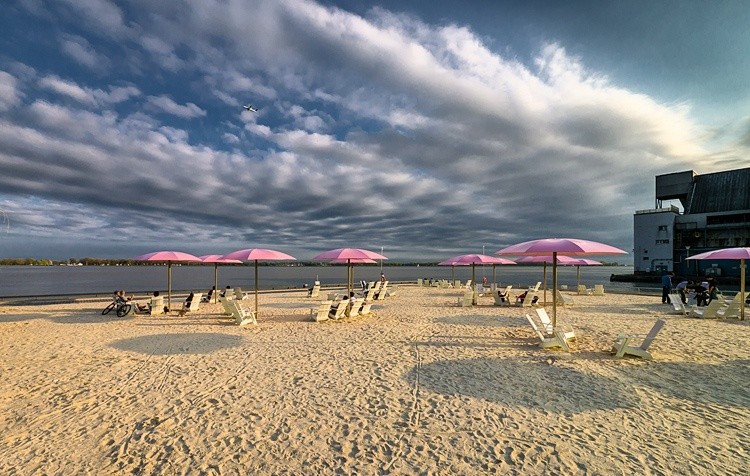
[242,104,258,112]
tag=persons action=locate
[706,276,719,305]
[221,285,230,297]
[676,280,692,305]
[114,289,146,309]
[330,296,348,315]
[695,285,708,306]
[186,293,194,308]
[206,286,215,300]
[366,280,379,300]
[661,273,674,304]
[350,292,355,310]
[519,291,527,303]
[498,291,507,302]
[150,291,159,304]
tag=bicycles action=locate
[102,292,131,317]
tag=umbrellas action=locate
[438,254,517,304]
[313,248,388,300]
[329,257,378,291]
[516,254,574,306]
[684,247,750,321]
[131,248,244,312]
[567,258,603,295]
[217,247,297,320]
[495,238,628,331]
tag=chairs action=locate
[149,279,750,359]
